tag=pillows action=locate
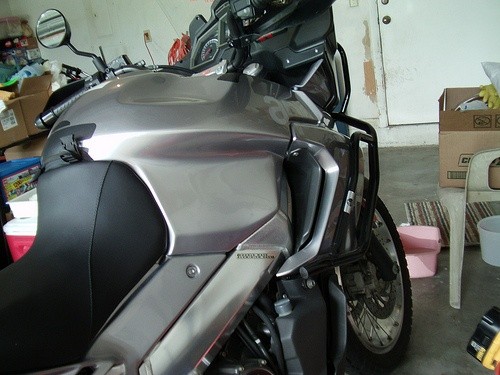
[482,62,499,97]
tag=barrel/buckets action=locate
[477,216,500,267]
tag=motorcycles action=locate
[0,0,414,375]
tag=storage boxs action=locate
[3,218,38,263]
[438,87,500,189]
[5,134,46,162]
[0,76,54,149]
[7,188,37,219]
[0,157,41,212]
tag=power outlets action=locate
[144,30,151,42]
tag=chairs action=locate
[441,149,500,310]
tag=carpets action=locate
[404,202,493,247]
